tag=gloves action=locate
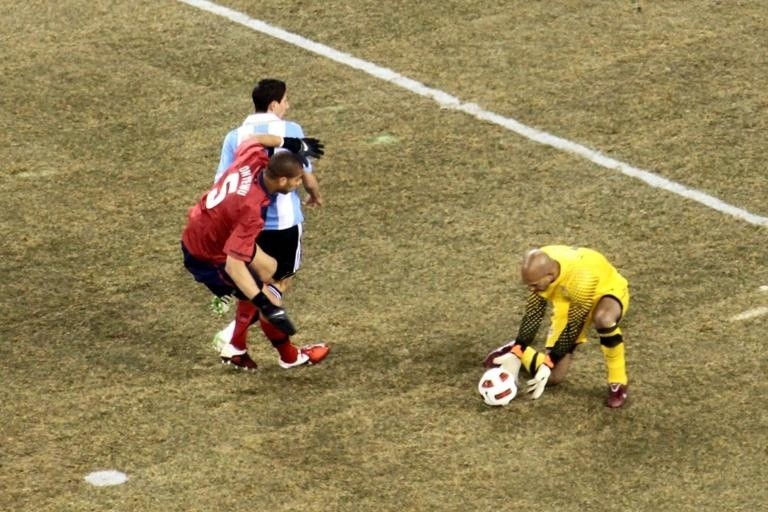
[492,352,522,384]
[527,364,552,399]
[251,291,296,336]
[280,137,324,168]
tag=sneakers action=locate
[608,382,627,408]
[212,330,258,372]
[211,295,232,314]
[279,343,330,369]
[485,340,517,368]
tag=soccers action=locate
[478,368,517,407]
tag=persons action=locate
[214,79,323,352]
[182,133,330,370]
[482,245,630,408]
